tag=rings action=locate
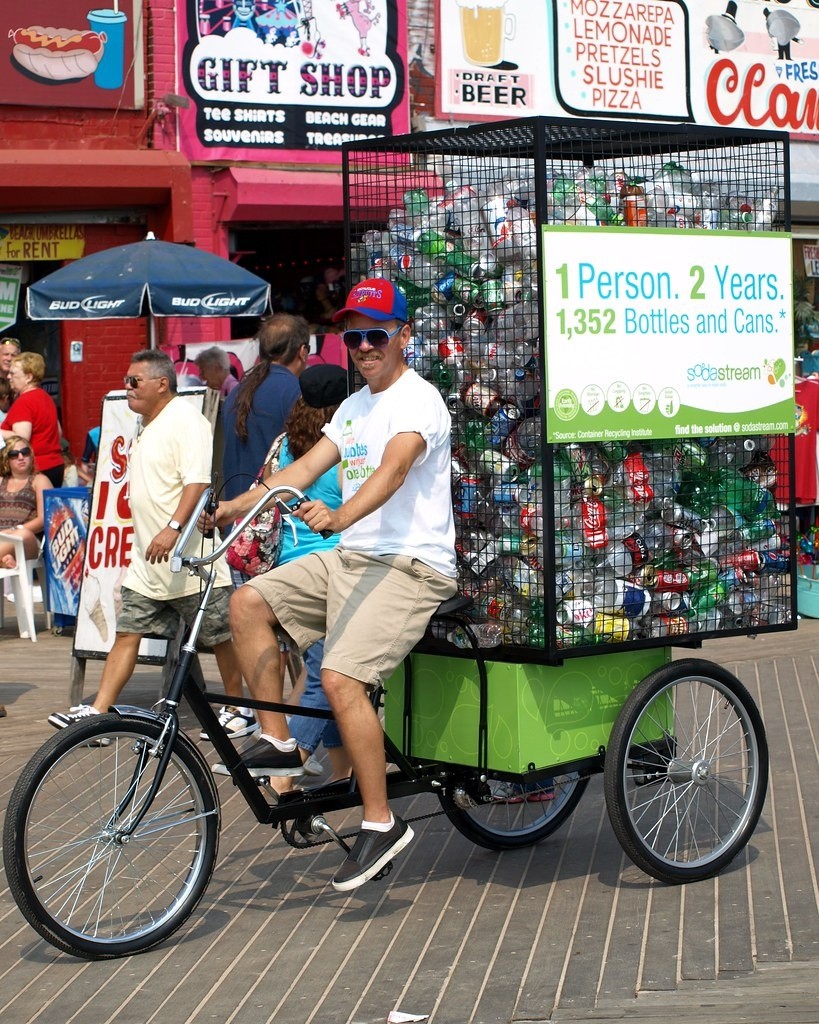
[164,552,168,556]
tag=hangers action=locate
[795,375,819,393]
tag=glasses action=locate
[7,447,31,459]
[0,338,20,347]
[338,325,403,349]
[122,376,161,388]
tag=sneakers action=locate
[210,735,304,778]
[332,812,414,891]
[303,755,323,775]
[491,778,554,801]
[200,705,259,739]
[48,704,110,746]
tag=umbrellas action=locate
[24,230,274,351]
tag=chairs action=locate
[0,532,51,643]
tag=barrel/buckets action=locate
[797,564,819,618]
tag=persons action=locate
[221,313,312,590]
[302,277,341,328]
[193,345,240,399]
[260,363,351,792]
[0,336,101,570]
[0,435,55,570]
[45,347,260,746]
[0,352,66,488]
[209,276,462,891]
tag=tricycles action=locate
[0,468,774,964]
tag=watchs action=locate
[166,519,183,534]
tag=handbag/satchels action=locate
[225,466,282,575]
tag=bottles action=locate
[351,157,791,646]
[43,496,88,615]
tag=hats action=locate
[333,279,407,321]
[299,364,348,408]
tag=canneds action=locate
[365,168,789,633]
